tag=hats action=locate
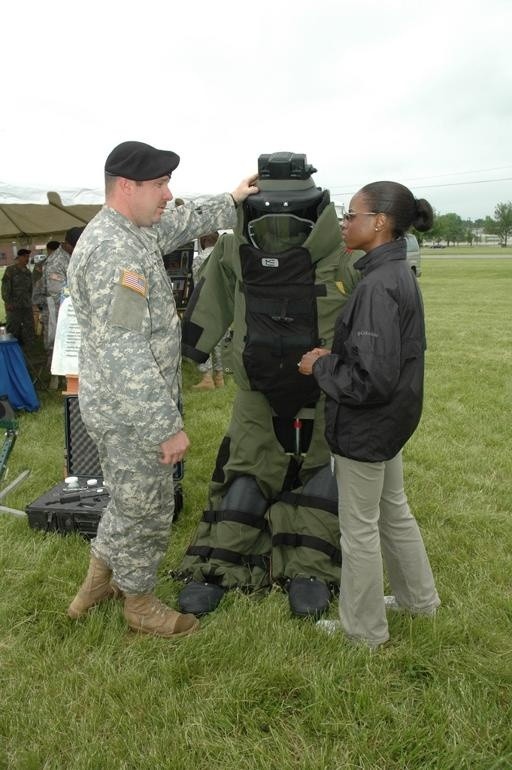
[16,248,32,253]
[46,241,59,247]
[65,227,84,247]
[105,141,180,181]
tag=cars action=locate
[430,243,448,248]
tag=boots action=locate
[68,554,114,620]
[192,372,224,390]
[121,592,201,637]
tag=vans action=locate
[401,232,423,278]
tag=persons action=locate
[1,227,84,434]
[297,181,440,650]
[68,141,260,637]
[193,231,223,390]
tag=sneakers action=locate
[384,596,437,614]
[316,619,378,652]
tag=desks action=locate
[0,334,41,412]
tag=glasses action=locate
[340,206,388,222]
